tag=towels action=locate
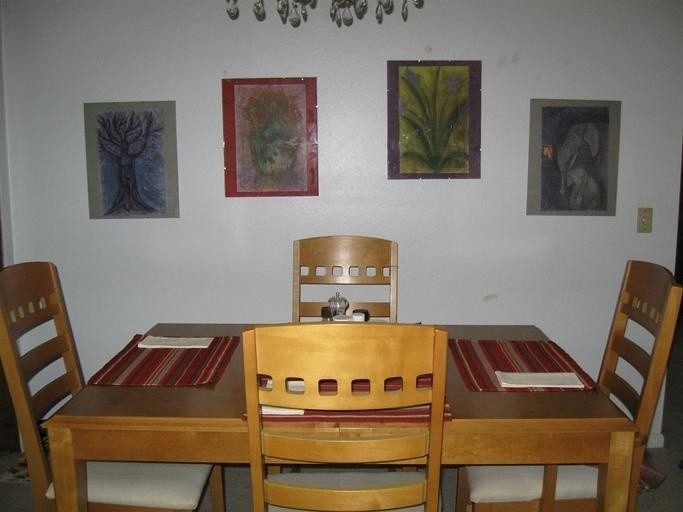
[138,335,214,349]
[495,370,584,388]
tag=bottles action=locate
[327,291,349,321]
[320,307,332,322]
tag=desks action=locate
[40,323,638,512]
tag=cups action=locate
[333,309,369,322]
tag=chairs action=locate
[456,260,683,511]
[243,322,447,512]
[292,236,398,322]
[0,261,226,512]
